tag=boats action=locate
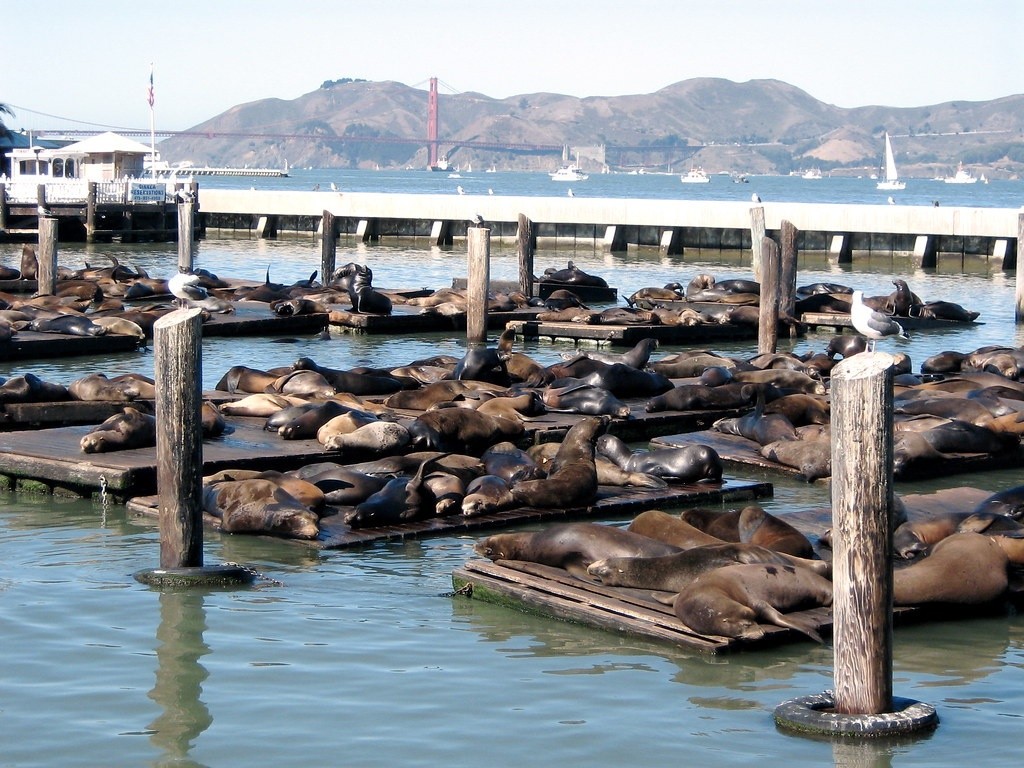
[802,169,823,179]
[931,176,944,181]
[945,160,977,184]
[550,172,589,182]
[680,168,710,184]
[548,165,584,176]
[427,155,455,172]
[718,171,731,175]
[628,169,646,175]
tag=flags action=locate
[147,70,154,108]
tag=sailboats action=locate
[877,131,907,189]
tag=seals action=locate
[0,241,1024,644]
